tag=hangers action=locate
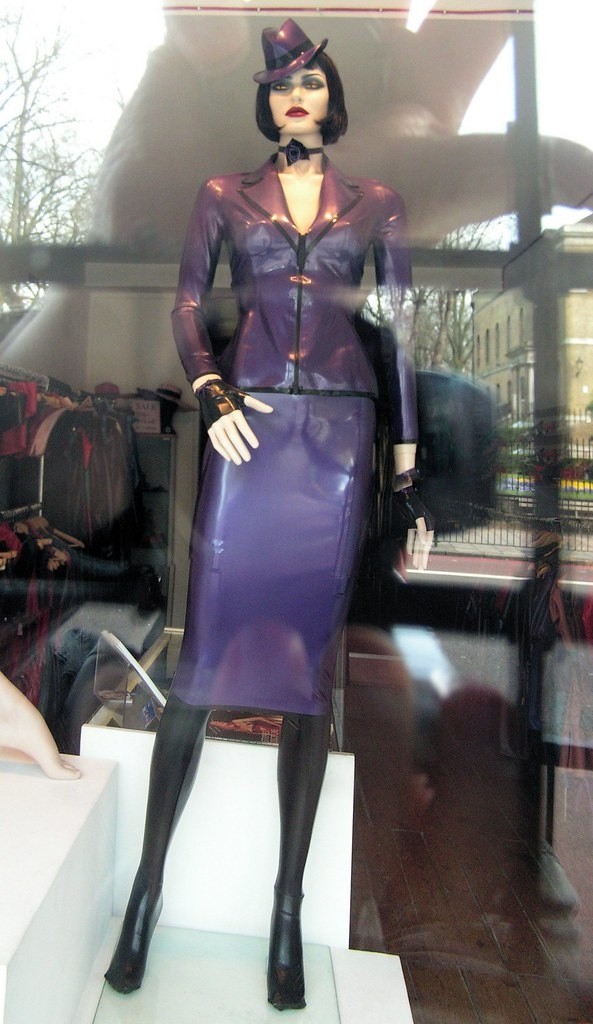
[0,362,117,413]
[0,503,84,571]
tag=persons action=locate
[104,18,435,1010]
[0,0,593,393]
